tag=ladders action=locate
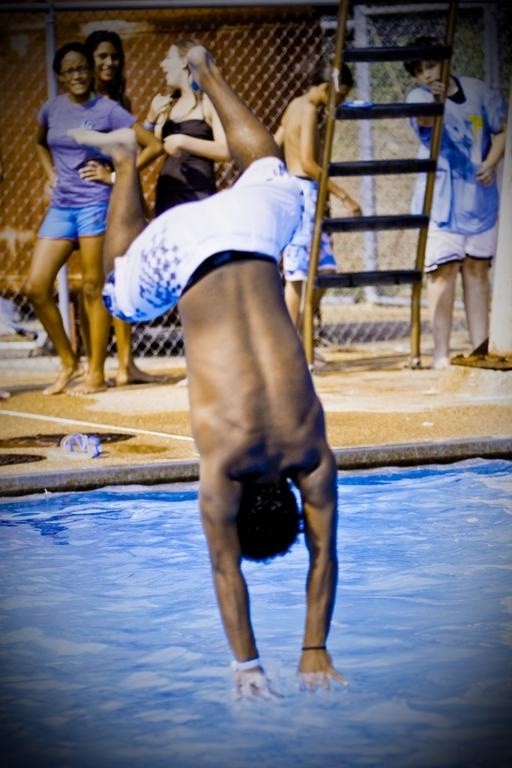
[303,0,456,373]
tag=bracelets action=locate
[301,644,327,650]
[142,117,156,130]
[235,657,260,671]
[108,169,117,185]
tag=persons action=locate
[24,40,163,395]
[141,40,232,215]
[402,35,508,375]
[66,42,350,701]
[82,28,167,386]
[272,54,367,334]
